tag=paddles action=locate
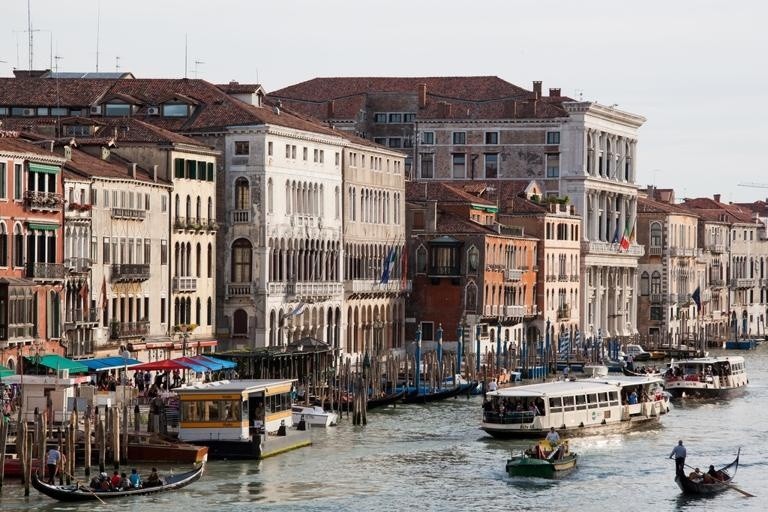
[59,468,105,504]
[671,458,755,496]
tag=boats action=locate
[171,378,313,459]
[627,345,653,359]
[664,356,750,398]
[481,375,672,437]
[290,403,337,427]
[298,366,521,411]
[506,449,578,480]
[675,449,741,497]
[33,461,203,501]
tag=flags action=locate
[612,217,638,251]
[693,287,702,313]
[379,240,409,288]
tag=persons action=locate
[254,401,265,420]
[546,427,560,448]
[0,384,21,422]
[671,440,686,474]
[481,378,542,420]
[298,383,305,401]
[690,465,721,485]
[624,354,731,407]
[44,447,163,493]
[99,368,182,391]
[563,366,570,377]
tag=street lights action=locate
[11,341,32,453]
[121,350,131,408]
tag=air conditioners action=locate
[90,106,101,115]
[23,109,34,116]
[148,108,159,115]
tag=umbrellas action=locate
[127,355,237,374]
